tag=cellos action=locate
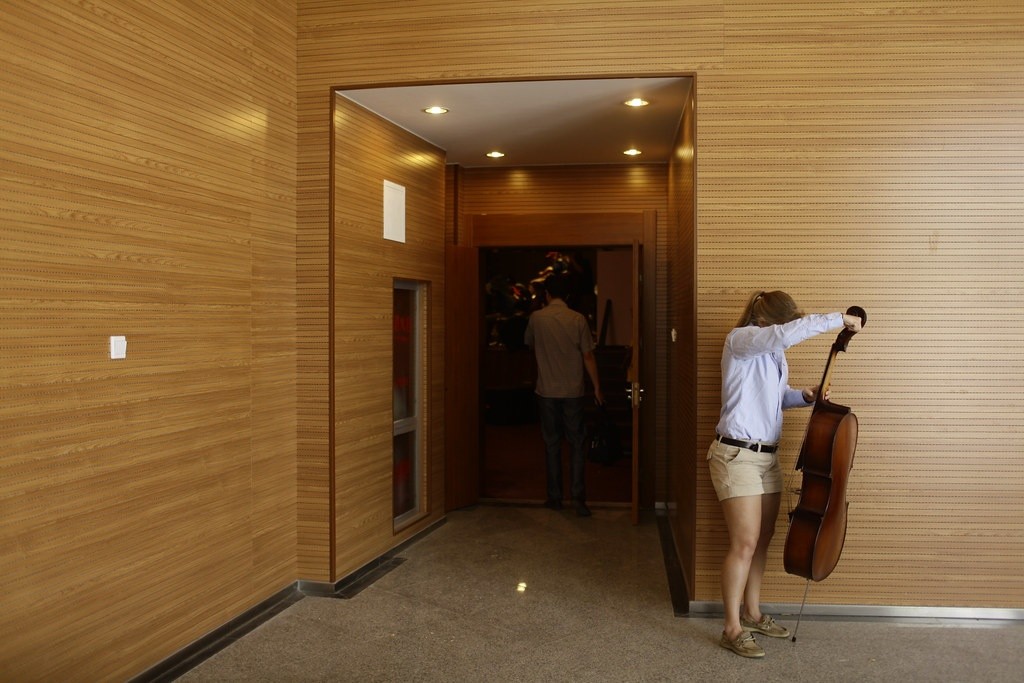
[781,305,868,645]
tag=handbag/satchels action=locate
[586,407,623,464]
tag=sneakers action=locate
[740,610,790,638]
[720,630,765,658]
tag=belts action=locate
[715,434,778,453]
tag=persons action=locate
[524,275,605,517]
[496,302,529,350]
[706,290,864,657]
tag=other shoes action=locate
[547,498,563,510]
[576,504,592,516]
